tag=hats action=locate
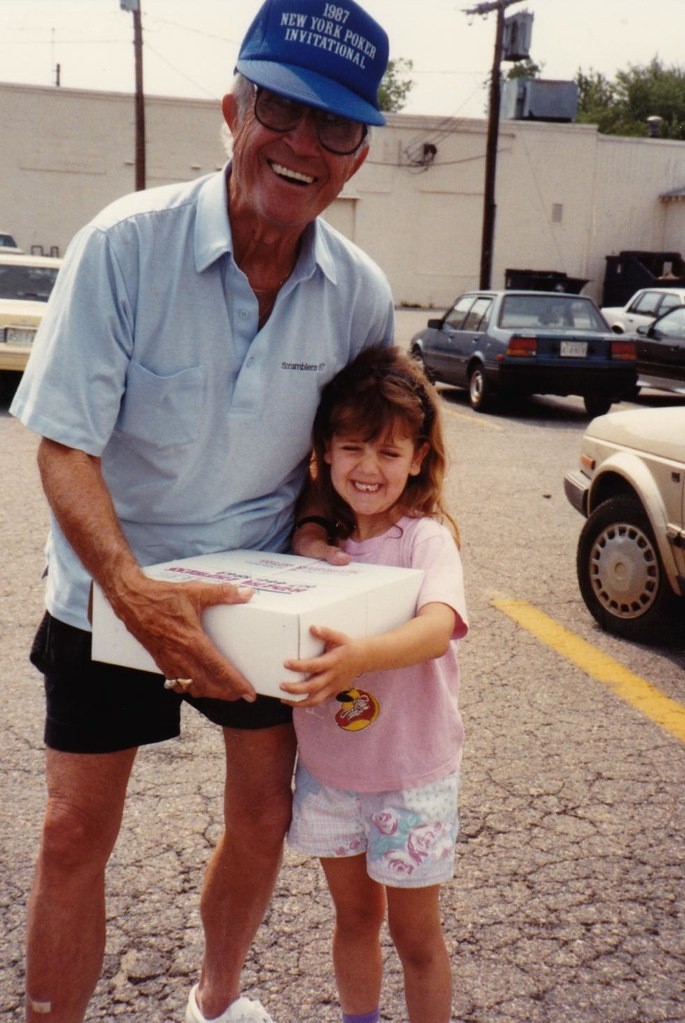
[235,0,385,126]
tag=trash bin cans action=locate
[505,269,590,315]
[601,251,685,307]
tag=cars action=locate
[0,232,24,256]
[620,303,685,395]
[564,405,685,646]
[410,290,641,419]
[601,289,685,339]
[0,253,62,372]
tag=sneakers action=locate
[184,980,274,1023]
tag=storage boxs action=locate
[88,547,424,700]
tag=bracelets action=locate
[291,516,335,541]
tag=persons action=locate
[280,344,470,1023]
[8,0,394,1023]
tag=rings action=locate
[164,678,192,690]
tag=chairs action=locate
[538,311,560,327]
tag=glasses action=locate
[252,82,368,156]
